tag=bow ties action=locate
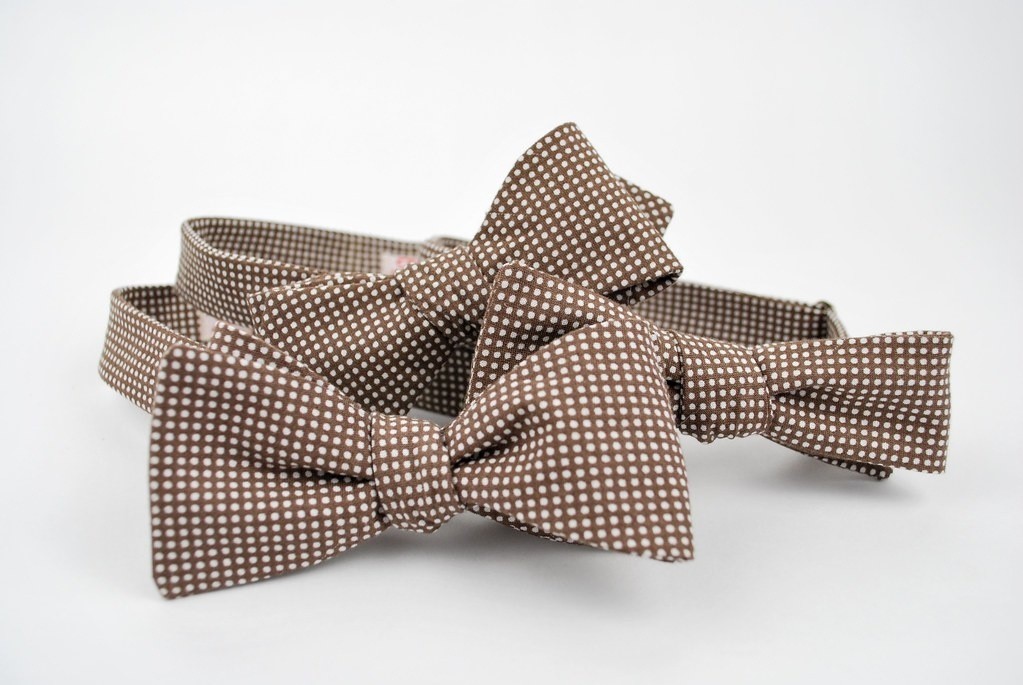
[99,282,699,602]
[180,123,846,420]
[455,262,951,485]
